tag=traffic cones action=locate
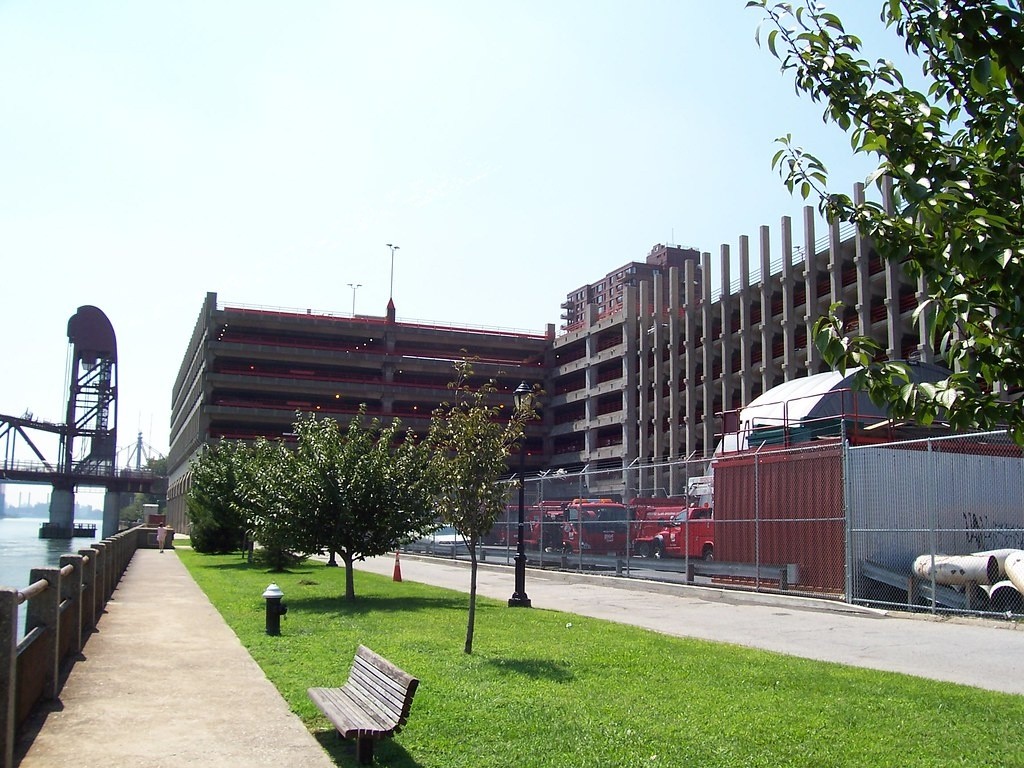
[392,551,402,581]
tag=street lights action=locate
[507,379,536,606]
[386,244,400,322]
[347,284,362,318]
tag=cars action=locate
[409,522,472,555]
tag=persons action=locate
[156,521,167,554]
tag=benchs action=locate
[307,644,420,764]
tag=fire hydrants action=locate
[263,581,288,636]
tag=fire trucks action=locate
[543,499,629,560]
[481,505,566,545]
[629,497,687,557]
[652,502,715,561]
